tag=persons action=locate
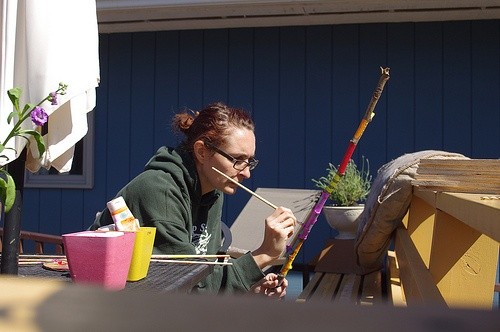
[83,101,294,305]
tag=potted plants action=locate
[311,159,372,240]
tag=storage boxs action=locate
[61,226,156,291]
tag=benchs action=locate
[298,273,381,305]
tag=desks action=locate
[15,258,215,293]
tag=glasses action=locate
[211,145,259,172]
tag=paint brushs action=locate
[148,259,233,266]
[151,255,232,258]
[18,253,67,267]
[210,167,306,227]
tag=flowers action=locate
[0,82,68,212]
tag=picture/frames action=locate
[24,111,94,189]
